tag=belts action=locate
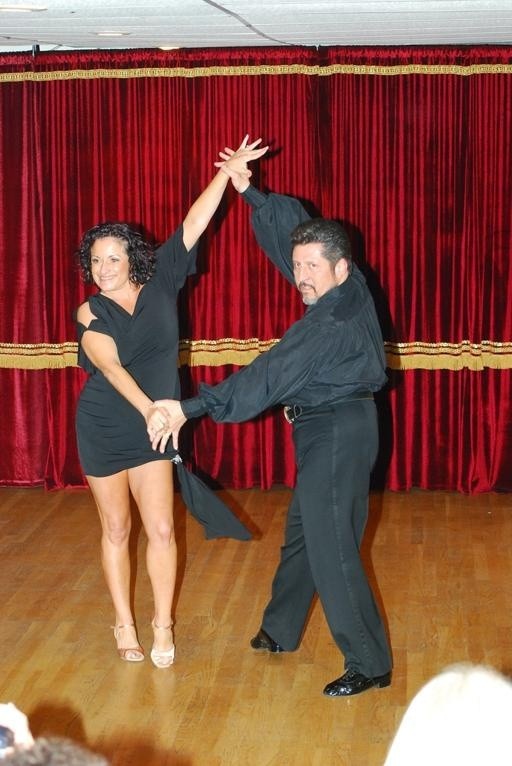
[285,390,374,419]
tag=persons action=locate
[0,703,111,766]
[76,132,269,667]
[151,144,394,697]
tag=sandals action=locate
[114,618,175,668]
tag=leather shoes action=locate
[324,667,391,696]
[251,628,286,652]
